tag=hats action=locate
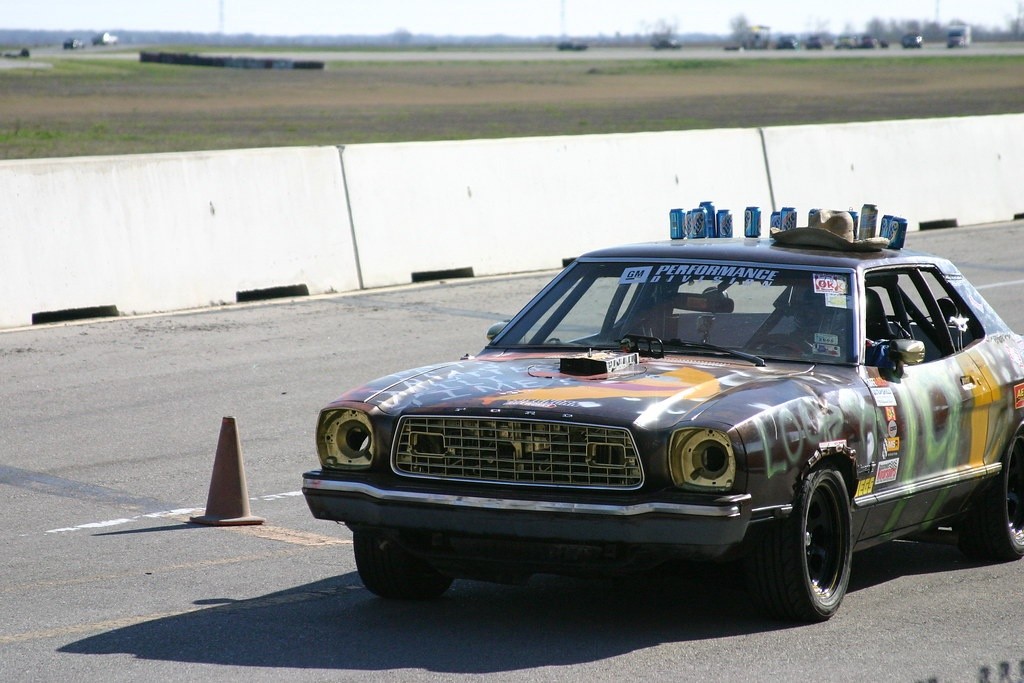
[771,209,890,251]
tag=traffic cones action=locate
[186,415,265,526]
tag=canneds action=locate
[669,201,908,250]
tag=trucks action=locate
[946,26,971,48]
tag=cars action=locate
[300,237,1022,616]
[657,38,682,49]
[556,39,590,51]
[725,25,891,51]
[901,32,922,49]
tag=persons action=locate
[768,291,904,382]
